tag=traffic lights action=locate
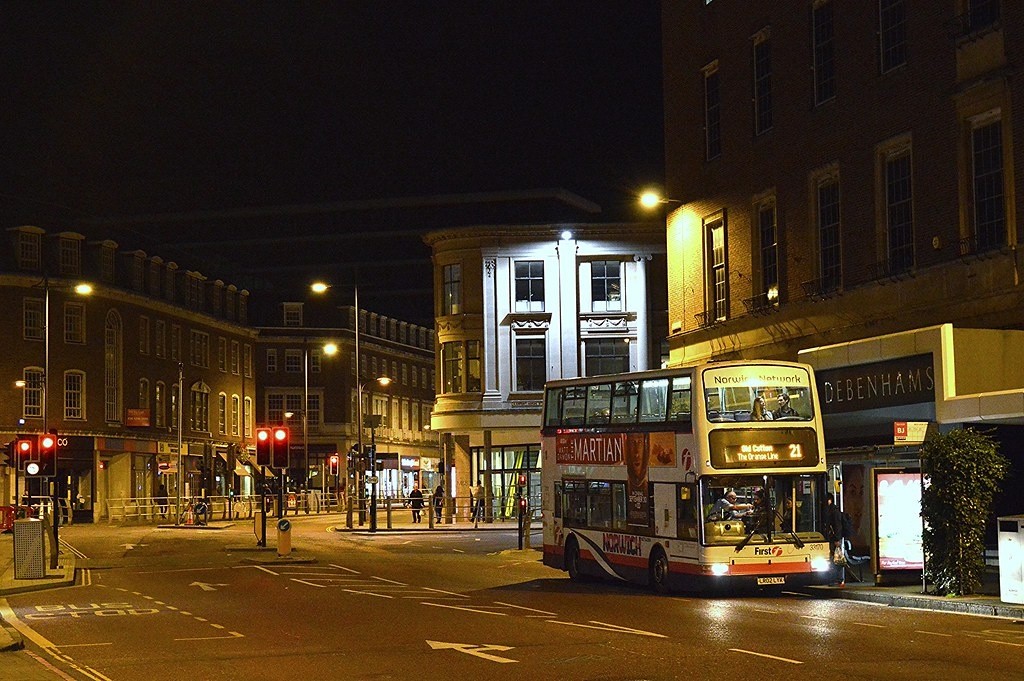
[18,440,32,471]
[520,499,526,510]
[272,426,291,469]
[519,475,527,487]
[256,428,271,466]
[25,460,40,479]
[330,456,338,476]
[38,434,57,479]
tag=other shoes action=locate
[436,520,440,524]
[840,581,845,587]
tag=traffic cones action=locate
[185,506,195,524]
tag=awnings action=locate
[217,452,250,476]
[246,455,276,477]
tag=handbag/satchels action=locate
[419,510,426,516]
[833,547,847,565]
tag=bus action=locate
[541,359,838,596]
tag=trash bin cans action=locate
[996,514,1024,606]
[13,517,46,580]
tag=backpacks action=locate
[834,507,855,537]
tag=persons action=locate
[157,484,168,519]
[842,464,868,547]
[772,393,799,421]
[754,489,764,513]
[710,491,754,521]
[779,496,802,533]
[627,432,649,496]
[432,486,445,524]
[406,483,425,524]
[468,479,485,523]
[263,484,272,513]
[832,534,847,588]
[750,396,773,420]
[826,494,842,557]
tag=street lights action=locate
[33,267,94,432]
[15,380,46,434]
[356,373,393,529]
[305,281,339,514]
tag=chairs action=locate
[548,409,752,428]
